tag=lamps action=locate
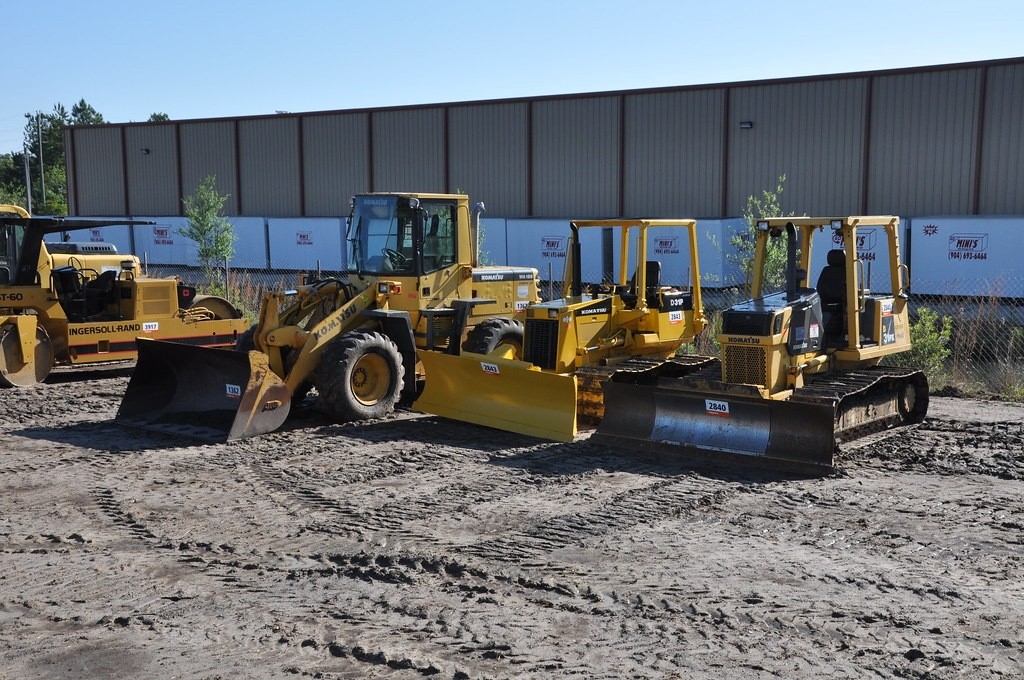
[140,149,149,154]
[739,120,753,129]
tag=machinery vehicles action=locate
[0,203,250,388]
[412,214,929,480]
[111,191,543,445]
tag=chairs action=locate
[85,271,116,297]
[412,233,441,272]
[816,249,847,310]
[619,261,662,305]
